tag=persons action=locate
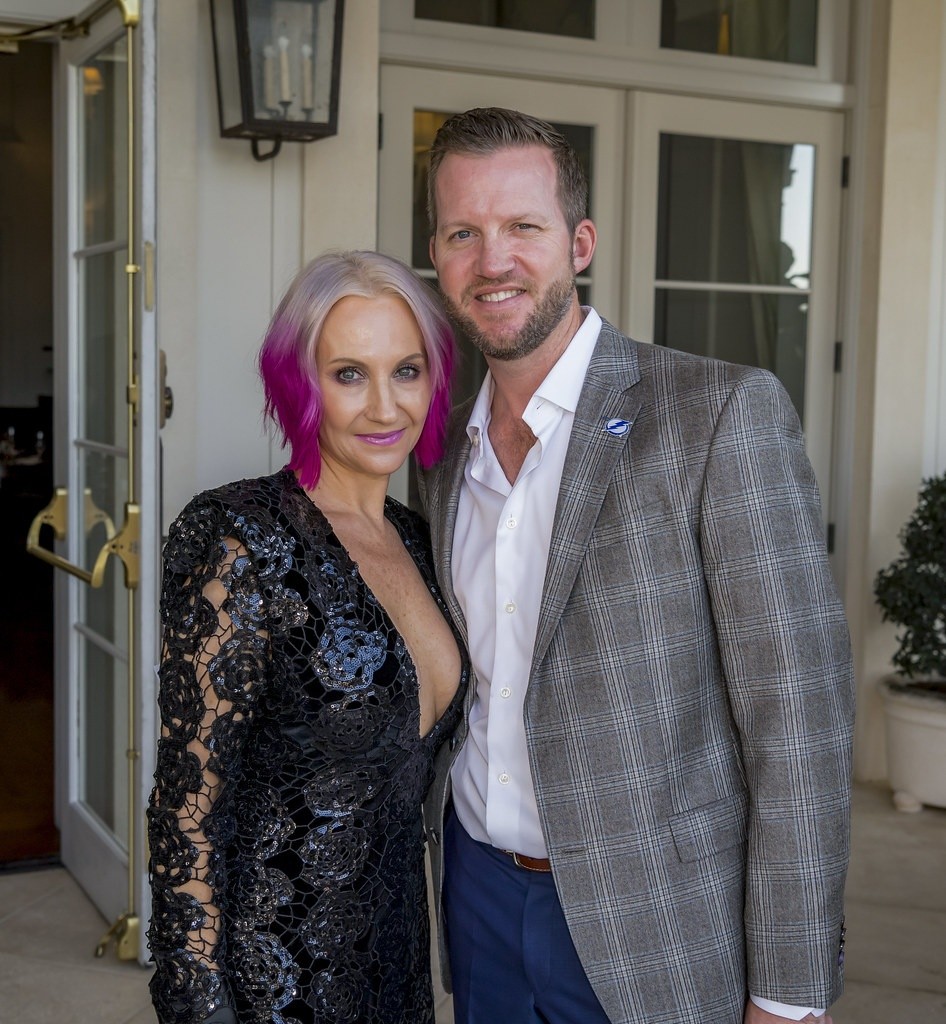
[410,106,857,1024]
[145,250,472,1022]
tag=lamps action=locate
[208,0,345,162]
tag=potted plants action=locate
[874,471,946,812]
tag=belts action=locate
[497,847,552,874]
